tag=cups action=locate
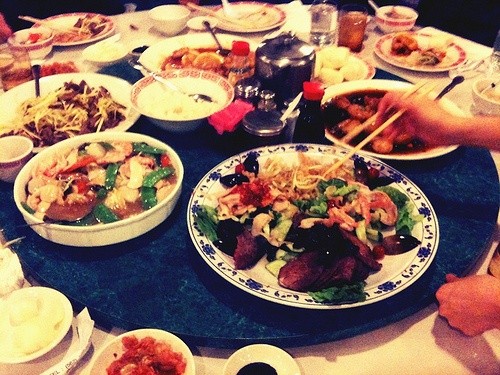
[309,0,339,48]
[0,46,34,92]
[336,3,369,54]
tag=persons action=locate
[378,91,500,337]
[122,0,200,14]
[0,14,13,44]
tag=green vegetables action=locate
[195,177,425,304]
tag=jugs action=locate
[253,31,318,108]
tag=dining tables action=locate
[0,5,500,375]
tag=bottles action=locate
[225,40,325,149]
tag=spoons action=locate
[127,57,214,104]
[0,200,94,234]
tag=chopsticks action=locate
[187,2,240,24]
[321,78,439,177]
[17,14,88,39]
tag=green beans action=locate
[23,141,176,225]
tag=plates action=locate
[208,1,287,35]
[135,32,264,78]
[185,142,439,309]
[0,72,140,154]
[30,12,117,49]
[208,1,288,34]
[312,47,376,88]
[374,31,468,73]
[187,16,219,31]
[0,287,301,375]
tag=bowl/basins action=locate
[129,67,236,133]
[0,135,34,182]
[320,77,466,160]
[375,5,419,34]
[0,4,192,68]
[13,131,185,248]
[471,77,500,116]
